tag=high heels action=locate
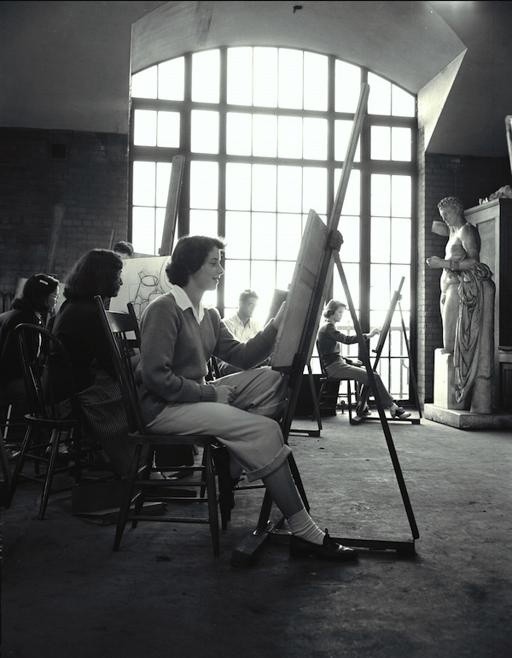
[391,408,411,419]
[357,406,372,415]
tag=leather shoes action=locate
[213,447,235,508]
[290,534,357,564]
[44,444,77,458]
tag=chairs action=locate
[1,322,89,526]
[314,338,362,424]
[95,292,233,562]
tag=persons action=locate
[217,288,262,375]
[427,195,494,414]
[141,236,359,560]
[317,298,413,419]
[4,241,134,418]
